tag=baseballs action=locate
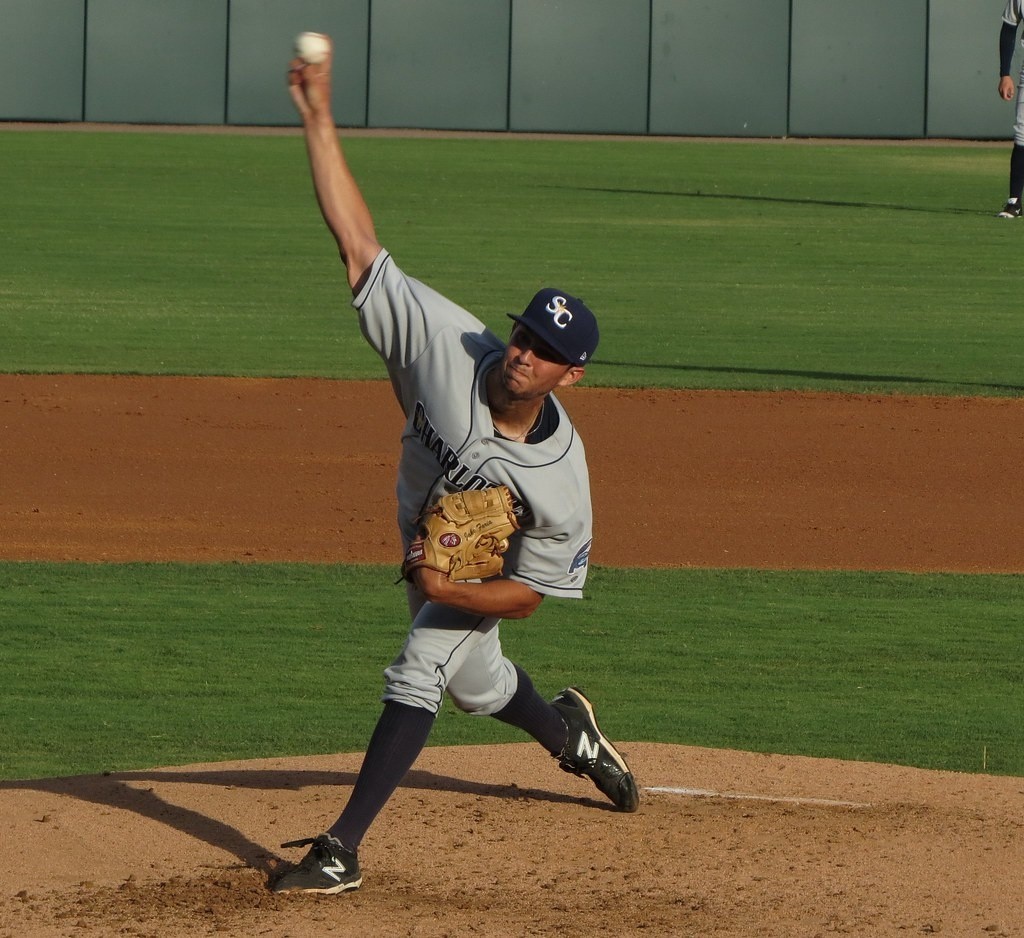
[295,31,330,64]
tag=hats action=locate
[506,286,597,368]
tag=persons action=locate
[999,0,1024,217]
[270,32,638,895]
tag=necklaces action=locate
[494,402,544,439]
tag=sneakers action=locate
[552,686,639,815]
[268,836,363,895]
[998,198,1022,218]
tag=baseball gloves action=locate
[391,483,522,587]
